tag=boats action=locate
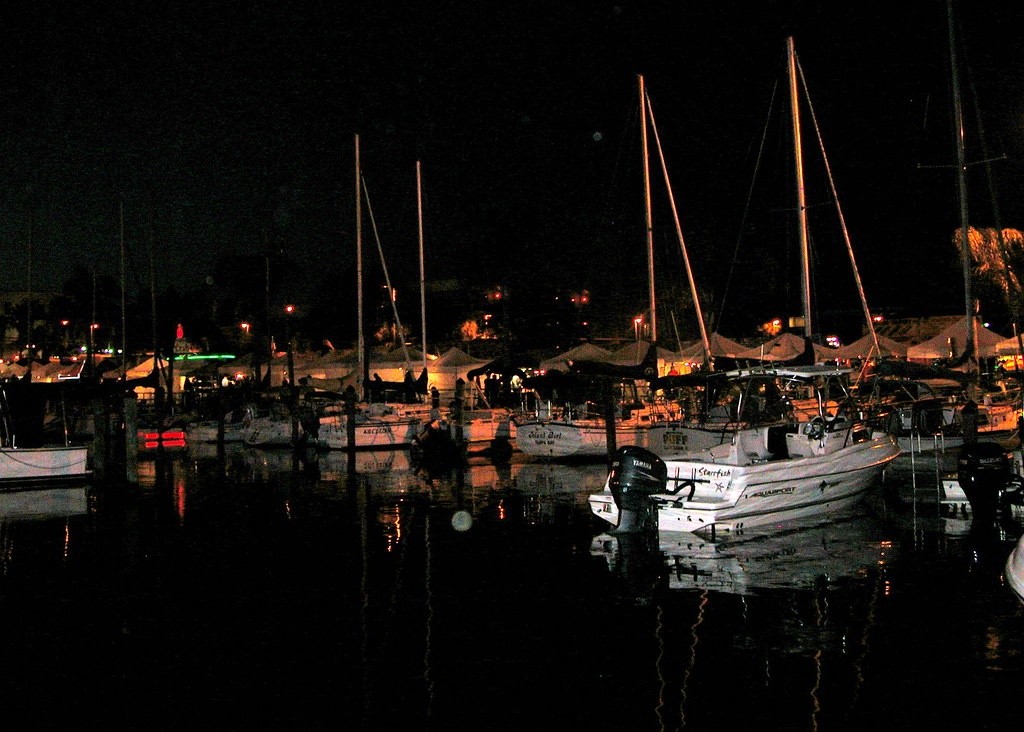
[0,444,94,484]
[582,362,905,534]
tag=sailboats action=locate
[0,1,1015,462]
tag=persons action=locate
[456,378,469,398]
[484,372,500,408]
[432,386,441,409]
[405,370,413,380]
[372,372,381,383]
[222,374,230,388]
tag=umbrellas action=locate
[538,314,1024,372]
[0,357,171,382]
[217,346,538,381]
